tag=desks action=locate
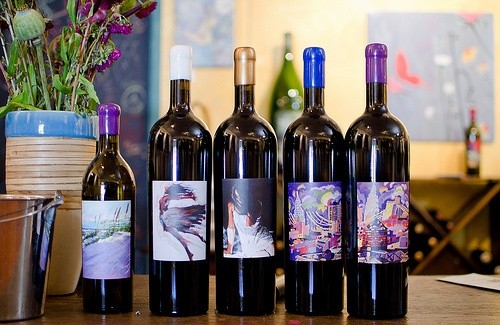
[0,274,500,325]
[408,174,500,277]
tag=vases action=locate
[4,109,100,296]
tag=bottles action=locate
[409,207,456,266]
[268,32,304,174]
[213,46,278,318]
[284,46,346,317]
[148,44,213,319]
[345,42,411,321]
[80,102,136,315]
[466,237,493,268]
[466,108,481,176]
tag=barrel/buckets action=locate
[0,188,64,321]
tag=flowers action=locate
[0,0,157,118]
